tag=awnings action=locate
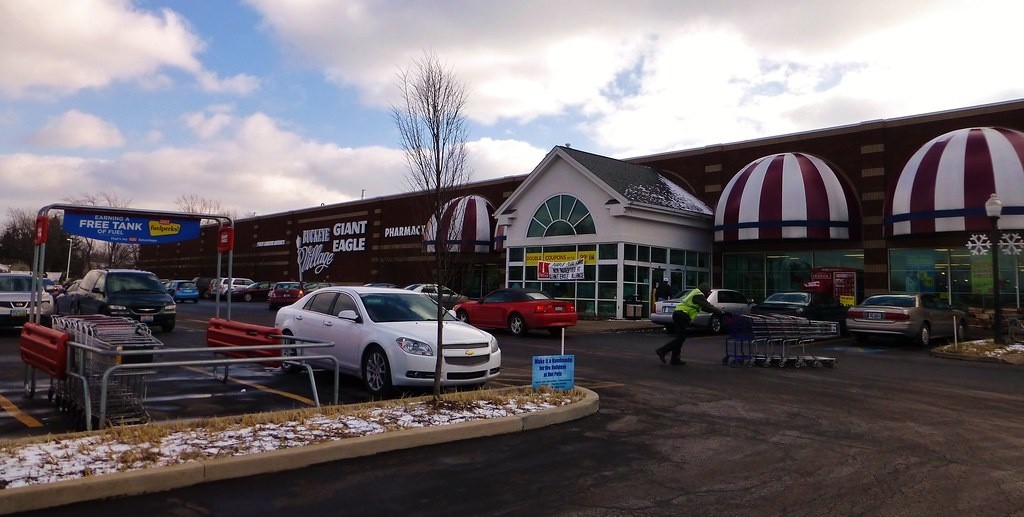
[421,195,507,253]
[888,127,1024,238]
[713,153,862,242]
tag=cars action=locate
[748,288,849,335]
[650,287,757,334]
[451,287,578,338]
[164,279,199,303]
[844,294,969,348]
[398,283,469,309]
[275,286,503,397]
[0,271,59,333]
[37,277,80,304]
[192,276,400,311]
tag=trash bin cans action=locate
[625,294,643,320]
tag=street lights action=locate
[985,193,1005,345]
[66,238,73,280]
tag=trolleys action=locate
[719,308,842,369]
[46,314,164,427]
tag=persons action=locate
[656,283,724,365]
[655,278,673,302]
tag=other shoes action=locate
[655,348,666,363]
[670,359,687,365]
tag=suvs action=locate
[74,268,177,332]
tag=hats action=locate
[663,278,668,281]
